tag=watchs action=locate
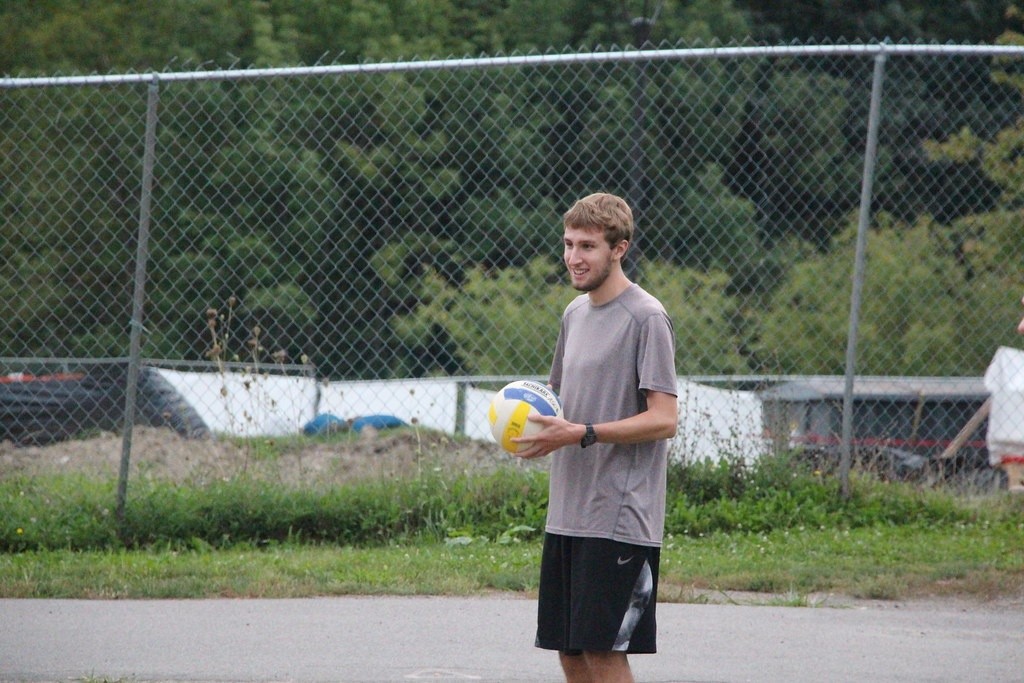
[581,422,597,449]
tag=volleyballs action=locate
[488,380,561,453]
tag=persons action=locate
[512,194,678,683]
